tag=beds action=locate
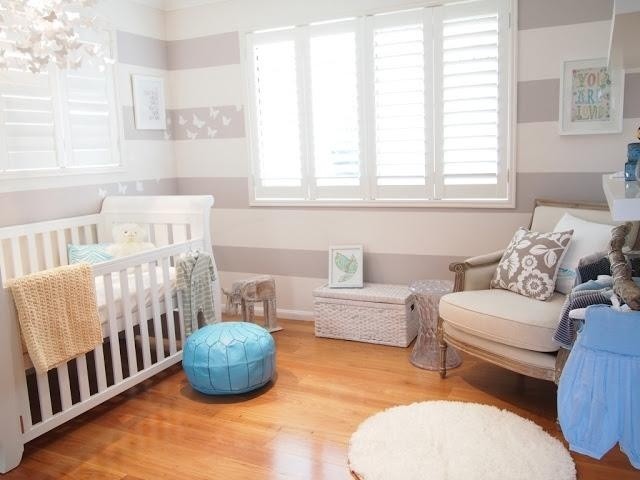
[0,194,222,474]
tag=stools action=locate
[409,279,463,371]
[182,321,277,396]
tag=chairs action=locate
[436,197,640,432]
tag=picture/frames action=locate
[328,244,364,288]
[557,57,625,136]
[132,73,168,132]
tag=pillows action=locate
[552,212,616,295]
[488,225,575,302]
[66,241,157,275]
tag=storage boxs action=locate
[313,281,419,348]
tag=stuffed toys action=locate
[103,223,157,276]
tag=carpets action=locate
[345,398,577,480]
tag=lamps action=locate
[0,0,117,76]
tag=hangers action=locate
[569,223,640,320]
[181,239,199,261]
